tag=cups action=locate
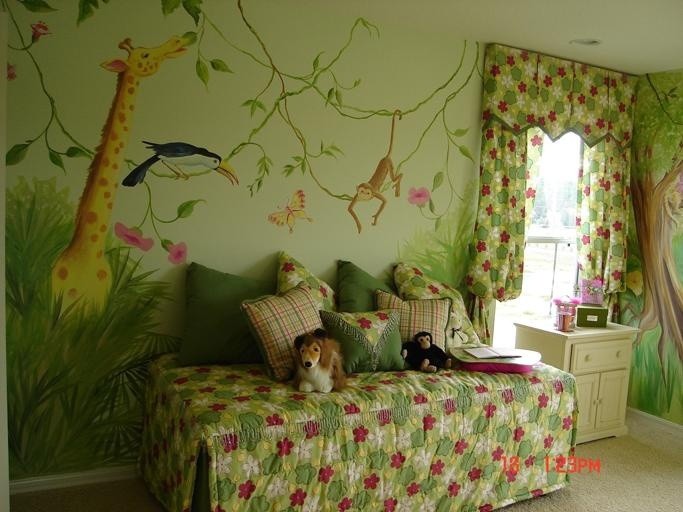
[557,312,576,332]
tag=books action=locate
[463,345,522,359]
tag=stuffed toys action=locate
[400,330,451,373]
[292,326,359,393]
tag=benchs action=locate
[136,351,580,512]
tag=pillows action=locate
[241,281,323,382]
[390,260,482,348]
[337,259,395,312]
[176,262,275,365]
[319,310,404,373]
[275,253,336,312]
[374,286,453,356]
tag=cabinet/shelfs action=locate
[514,320,642,448]
